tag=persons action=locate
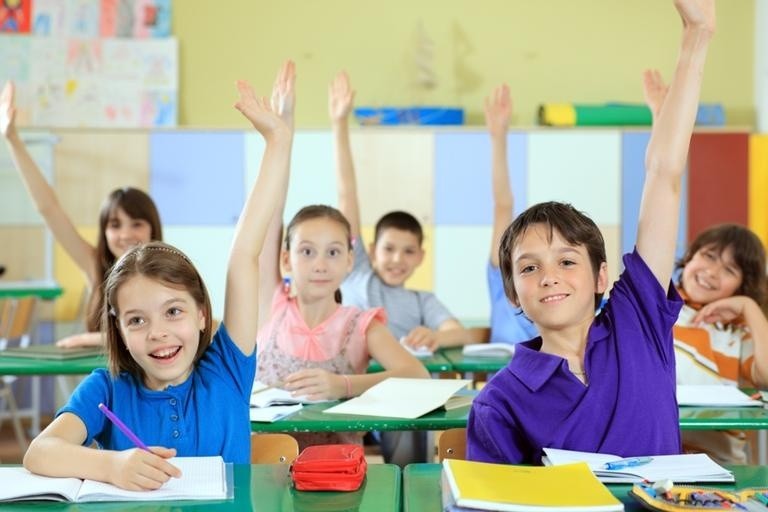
[1,74,166,352]
[249,57,432,462]
[323,69,478,464]
[636,63,768,464]
[479,83,545,382]
[19,77,296,495]
[461,0,721,465]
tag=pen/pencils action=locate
[603,456,653,470]
[98,404,151,452]
[635,482,768,510]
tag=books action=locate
[250,404,308,425]
[326,372,476,421]
[671,381,763,409]
[438,453,628,512]
[536,443,738,484]
[243,375,334,407]
[0,454,240,505]
[1,341,112,363]
